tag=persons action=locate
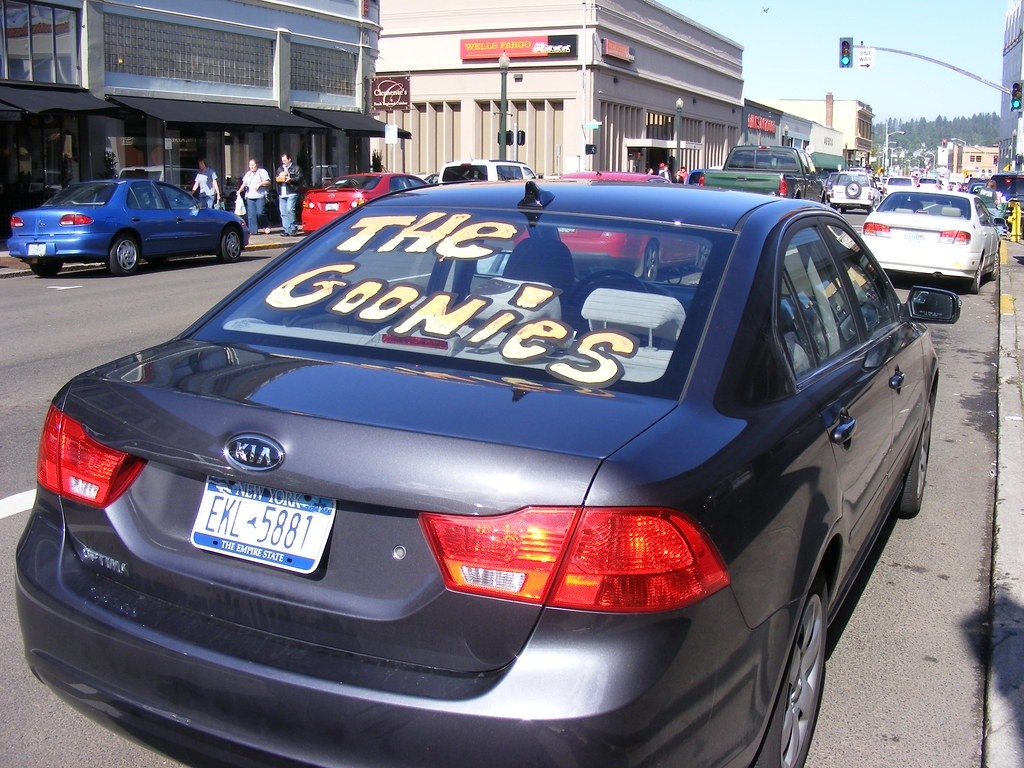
[647,168,653,175]
[237,159,272,235]
[677,166,688,180]
[947,185,967,192]
[915,177,920,187]
[977,179,1003,235]
[936,184,942,190]
[191,159,220,208]
[277,153,303,237]
[655,163,671,181]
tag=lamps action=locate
[514,74,522,79]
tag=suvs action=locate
[436,160,544,187]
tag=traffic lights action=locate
[1009,80,1024,113]
[516,130,527,147]
[838,37,854,68]
[585,144,597,155]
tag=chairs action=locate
[135,188,159,209]
[894,201,924,213]
[461,237,687,352]
[942,207,962,217]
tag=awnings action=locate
[108,95,332,135]
[810,152,846,170]
[293,109,411,139]
[0,82,121,122]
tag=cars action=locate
[422,173,439,184]
[10,178,962,767]
[300,172,430,235]
[685,168,719,186]
[510,170,711,283]
[5,177,252,278]
[859,185,1006,296]
[824,170,1024,215]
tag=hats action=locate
[659,163,665,167]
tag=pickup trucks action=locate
[698,143,827,206]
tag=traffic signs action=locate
[857,47,875,70]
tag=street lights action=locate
[889,146,903,173]
[782,123,790,146]
[674,97,686,172]
[1011,128,1018,171]
[884,120,906,180]
[1007,145,1013,172]
[881,141,898,176]
[494,49,514,160]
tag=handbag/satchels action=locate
[234,192,246,215]
[214,199,225,210]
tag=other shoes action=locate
[281,232,289,237]
[291,227,298,235]
[264,226,271,234]
